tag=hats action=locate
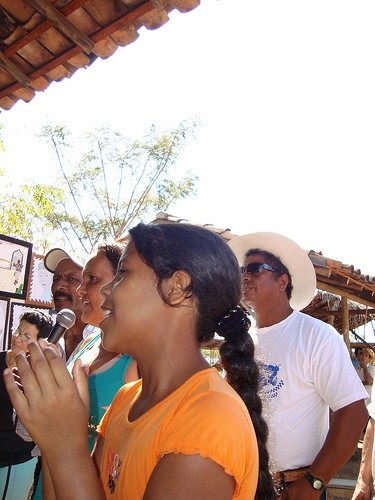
[226,232,316,311]
[358,348,373,359]
[45,247,95,273]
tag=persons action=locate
[31,243,143,500]
[351,345,375,500]
[0,310,54,500]
[223,232,370,500]
[2,221,279,500]
[42,246,101,367]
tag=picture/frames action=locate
[25,253,54,309]
[0,234,33,299]
[0,297,10,351]
[8,302,52,352]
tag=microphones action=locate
[47,309,76,344]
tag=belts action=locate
[271,469,311,482]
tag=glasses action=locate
[12,328,35,343]
[237,262,280,275]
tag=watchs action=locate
[303,471,327,492]
